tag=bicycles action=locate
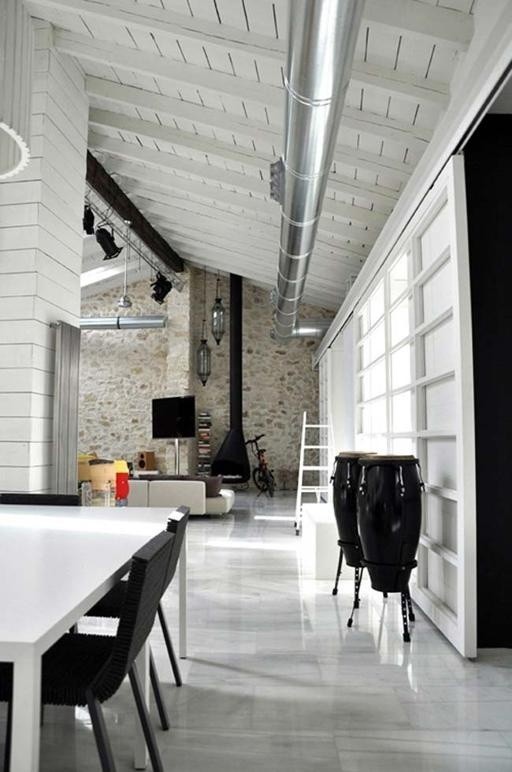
[244,433,277,498]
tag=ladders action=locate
[294,411,333,536]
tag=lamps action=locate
[83,205,174,312]
[194,265,227,388]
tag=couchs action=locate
[128,460,236,518]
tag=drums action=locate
[357,454,426,593]
[330,452,382,568]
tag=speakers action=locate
[137,451,155,470]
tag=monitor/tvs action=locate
[151,395,196,440]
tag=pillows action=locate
[138,473,223,497]
